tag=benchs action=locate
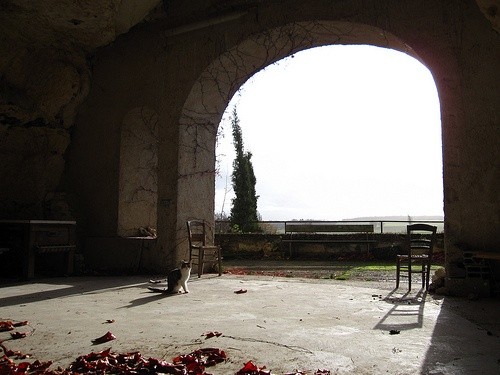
[282,225,376,261]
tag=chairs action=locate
[186,218,222,279]
[395,224,437,291]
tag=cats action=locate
[146,260,192,295]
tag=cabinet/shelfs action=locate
[0,220,77,281]
